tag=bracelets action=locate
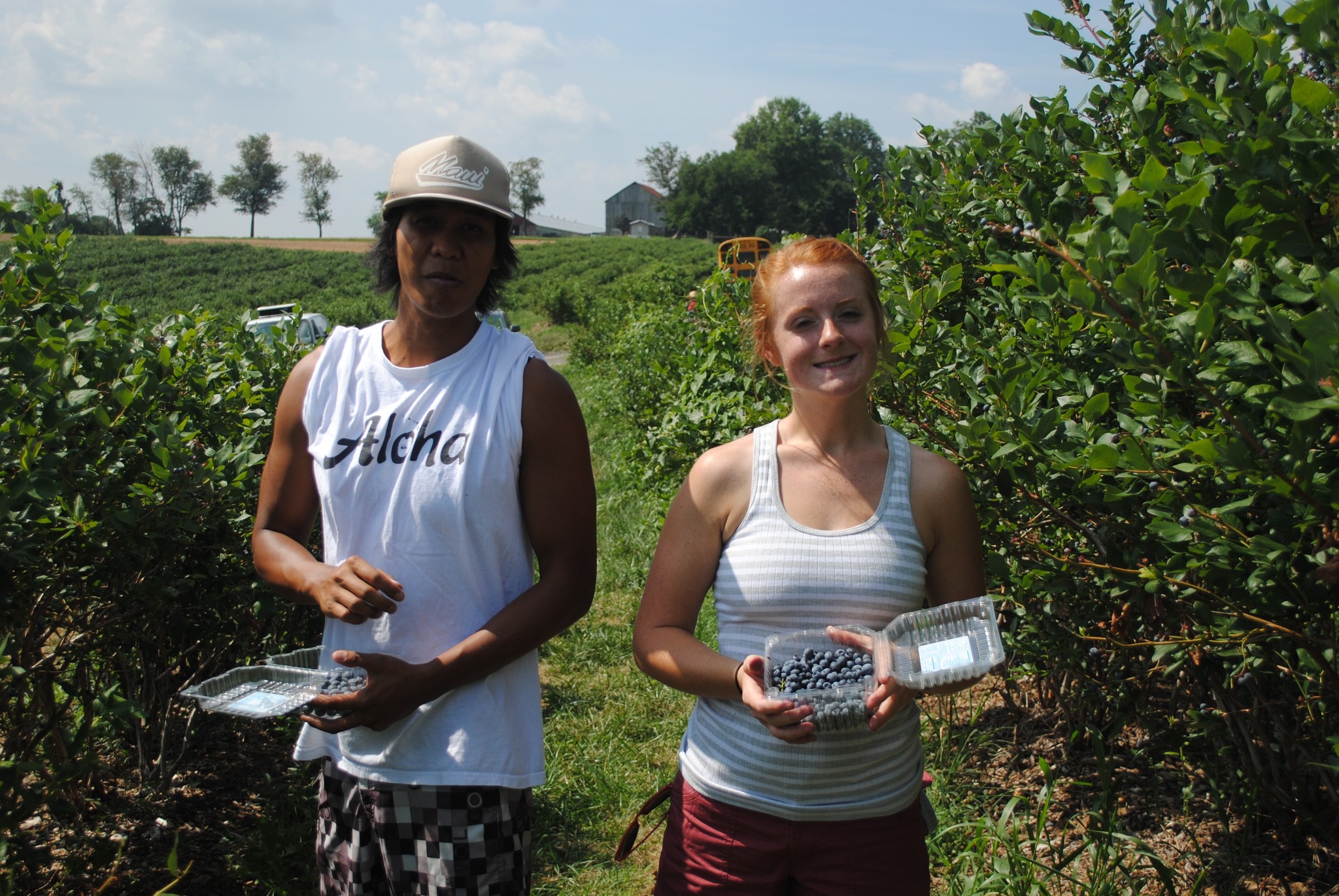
[734,659,745,704]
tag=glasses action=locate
[613,782,680,857]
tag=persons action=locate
[635,236,985,896]
[250,136,597,896]
[687,290,698,310]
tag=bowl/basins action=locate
[762,594,1006,735]
[178,645,368,720]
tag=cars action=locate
[243,303,330,355]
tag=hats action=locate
[379,136,521,224]
